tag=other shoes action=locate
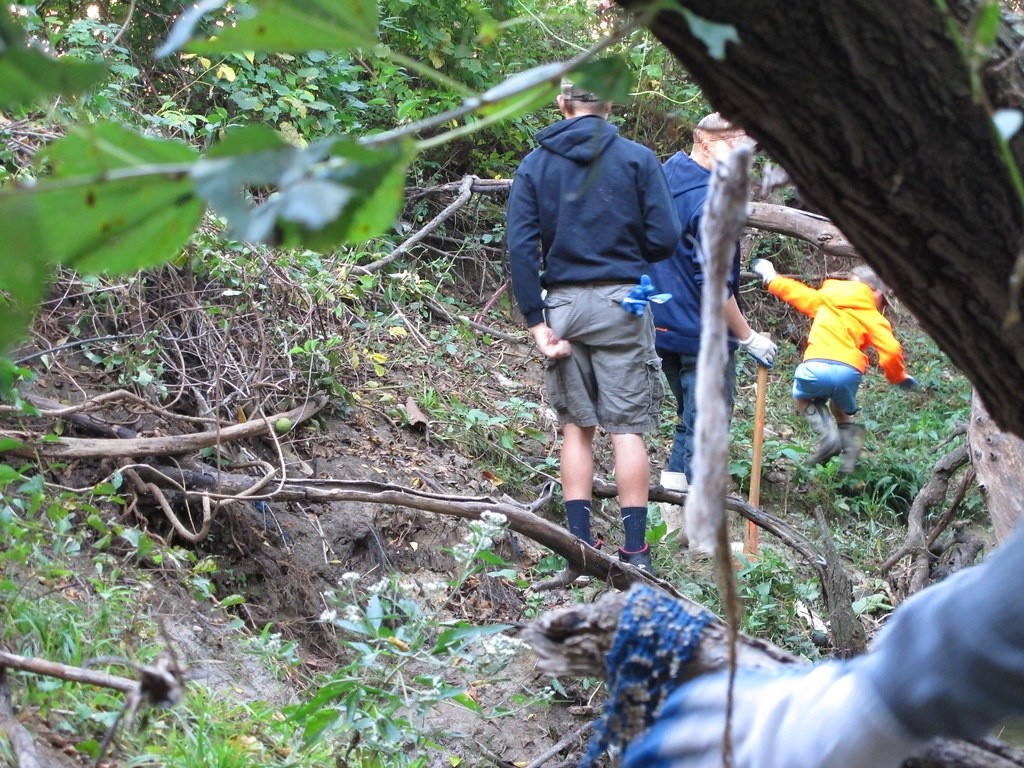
[591,538,603,551]
[618,542,655,577]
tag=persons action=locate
[749,258,923,490]
[649,112,779,545]
[507,75,683,578]
[624,515,1024,768]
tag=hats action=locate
[853,266,900,313]
[561,77,602,101]
[693,112,758,148]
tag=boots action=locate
[804,400,842,467]
[659,469,689,546]
[837,422,864,473]
[686,485,745,561]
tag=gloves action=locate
[900,375,927,394]
[621,275,673,317]
[751,258,777,289]
[623,663,928,768]
[738,329,778,367]
